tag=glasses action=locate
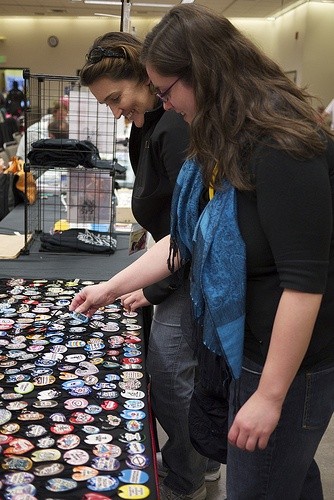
[156,68,189,103]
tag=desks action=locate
[0,197,160,500]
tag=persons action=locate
[69,2,334,500]
[80,32,221,500]
[322,99,334,132]
[0,80,73,176]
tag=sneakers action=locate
[157,451,221,499]
